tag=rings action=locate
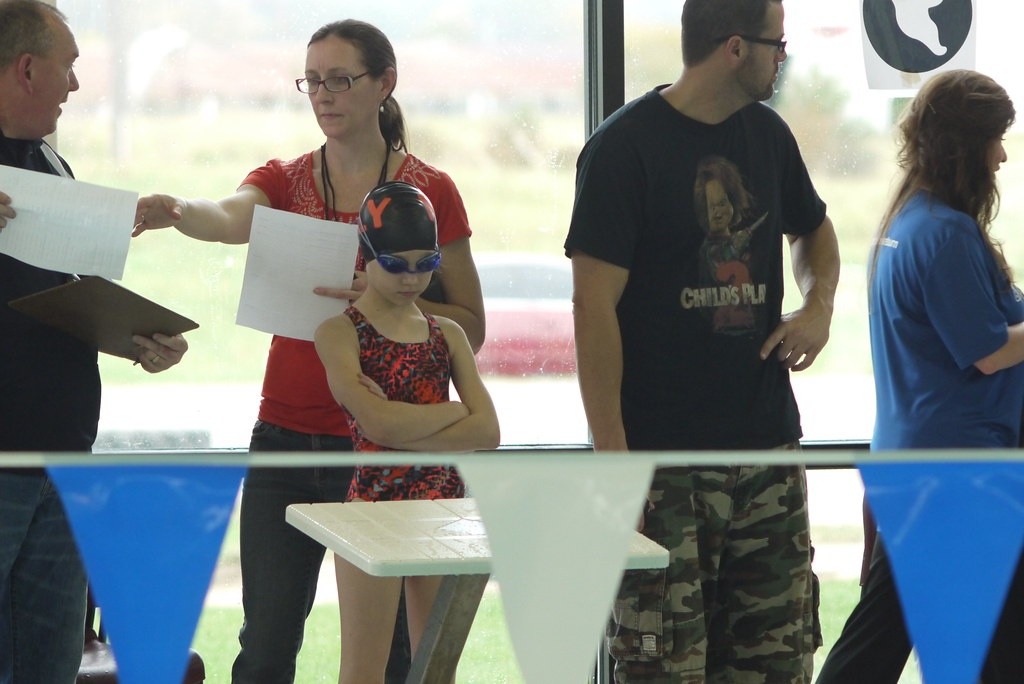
[152,355,160,364]
[141,215,145,222]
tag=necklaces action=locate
[320,142,390,225]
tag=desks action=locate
[285,499,670,684]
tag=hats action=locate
[359,183,438,262]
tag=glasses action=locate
[296,70,370,94]
[717,34,787,53]
[362,234,441,274]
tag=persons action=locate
[0,0,189,684]
[133,19,487,684]
[565,0,839,684]
[814,69,1024,684]
[314,179,504,684]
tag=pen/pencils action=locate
[132,360,140,366]
